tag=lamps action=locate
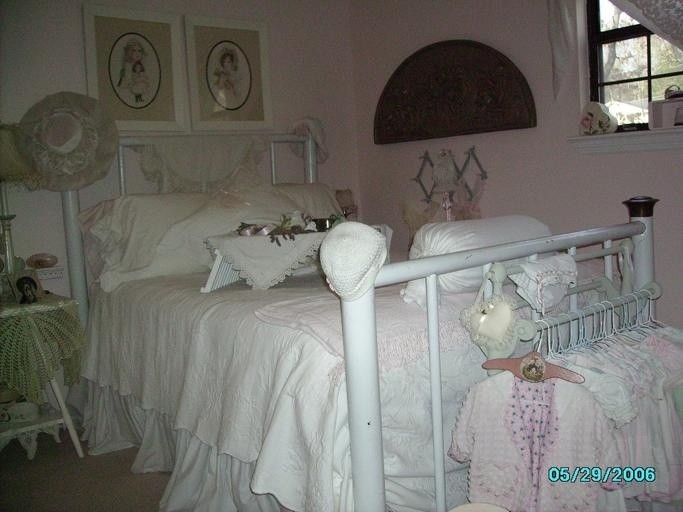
[0,122,46,279]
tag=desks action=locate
[0,293,88,461]
[200,221,394,298]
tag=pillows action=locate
[399,212,551,308]
[75,183,358,280]
[156,163,312,270]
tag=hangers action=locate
[479,287,667,383]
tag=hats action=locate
[15,90,120,194]
[320,221,391,301]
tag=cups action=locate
[323,219,332,229]
[281,210,307,232]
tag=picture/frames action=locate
[183,12,275,130]
[79,0,191,135]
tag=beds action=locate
[60,127,659,511]
